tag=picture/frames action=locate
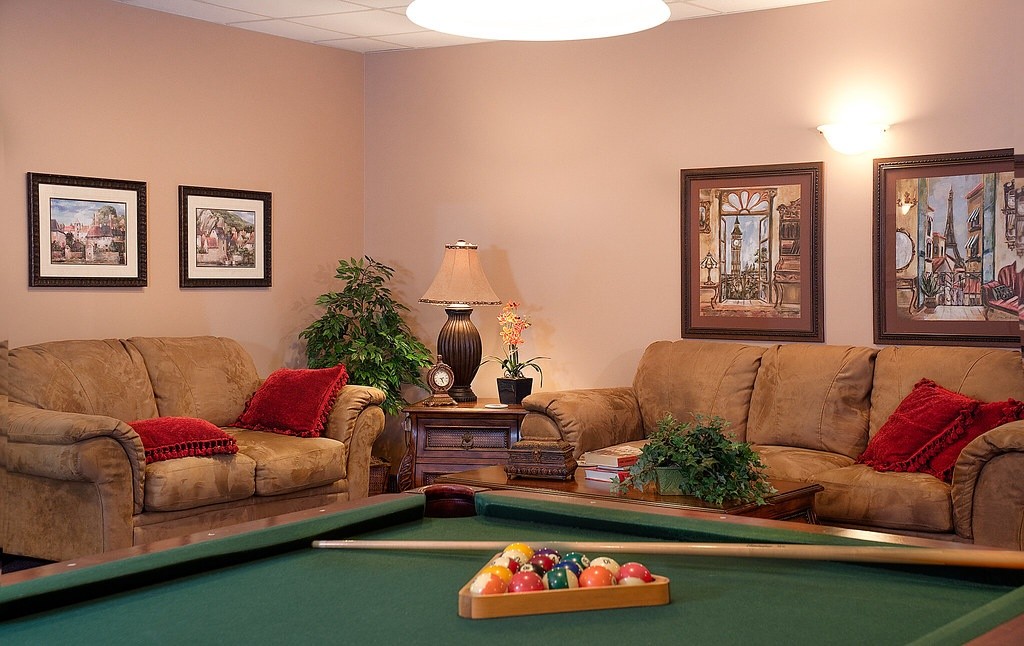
[178,184,273,288]
[680,162,824,342]
[872,148,1024,348]
[26,171,147,288]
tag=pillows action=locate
[857,377,1024,482]
[228,361,349,439]
[128,417,238,464]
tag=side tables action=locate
[398,395,529,493]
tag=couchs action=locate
[1,335,387,561]
[521,340,1023,551]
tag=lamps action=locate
[418,239,502,402]
[405,0,671,42]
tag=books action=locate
[584,444,645,467]
[596,465,631,470]
[584,469,629,483]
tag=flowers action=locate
[471,300,550,388]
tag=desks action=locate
[0,482,1024,646]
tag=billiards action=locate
[470,541,653,596]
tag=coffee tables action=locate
[436,464,824,525]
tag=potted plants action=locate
[298,256,435,497]
[610,410,778,507]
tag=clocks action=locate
[425,354,458,405]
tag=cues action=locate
[310,534,1024,572]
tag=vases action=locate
[496,377,532,405]
